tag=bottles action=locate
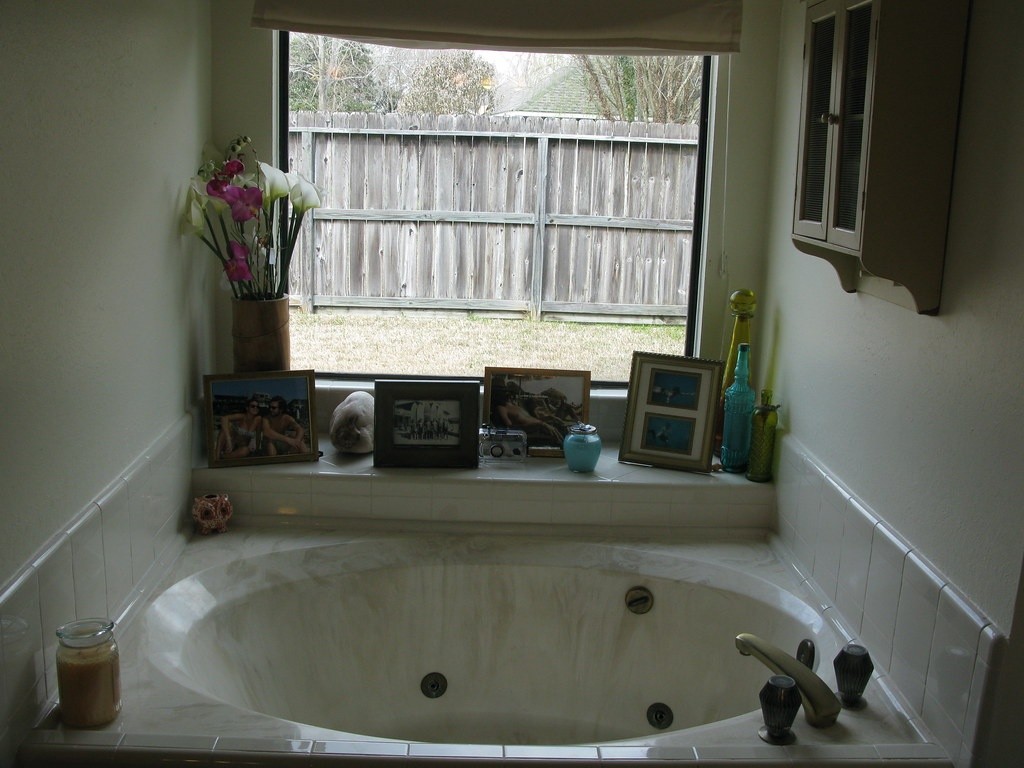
[562,421,602,474]
[720,342,756,475]
[55,617,122,728]
[745,388,778,483]
[715,288,755,457]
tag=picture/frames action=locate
[482,366,591,458]
[374,379,480,469]
[619,351,725,474]
[202,369,319,468]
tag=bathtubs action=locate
[130,528,847,762]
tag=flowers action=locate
[185,133,321,301]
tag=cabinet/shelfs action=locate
[791,0,968,315]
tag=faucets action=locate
[732,630,843,730]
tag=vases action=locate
[231,292,291,371]
